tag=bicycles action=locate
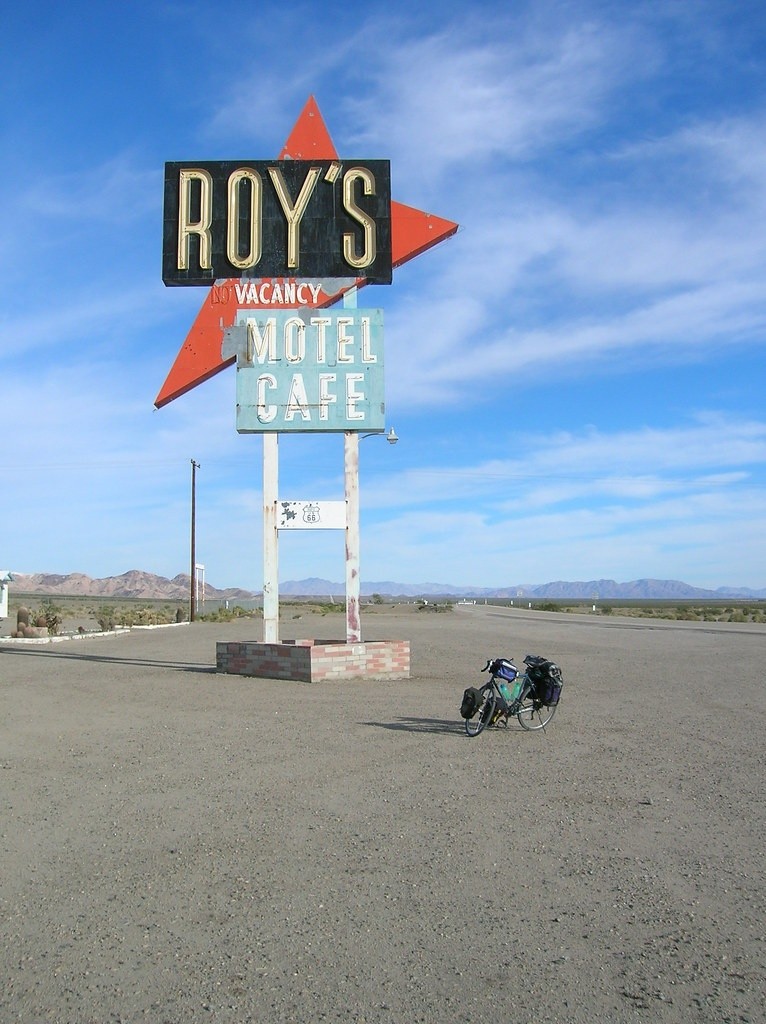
[465,658,562,737]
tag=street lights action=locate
[188,459,201,623]
[343,426,399,642]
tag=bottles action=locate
[500,684,511,701]
[511,683,521,702]
